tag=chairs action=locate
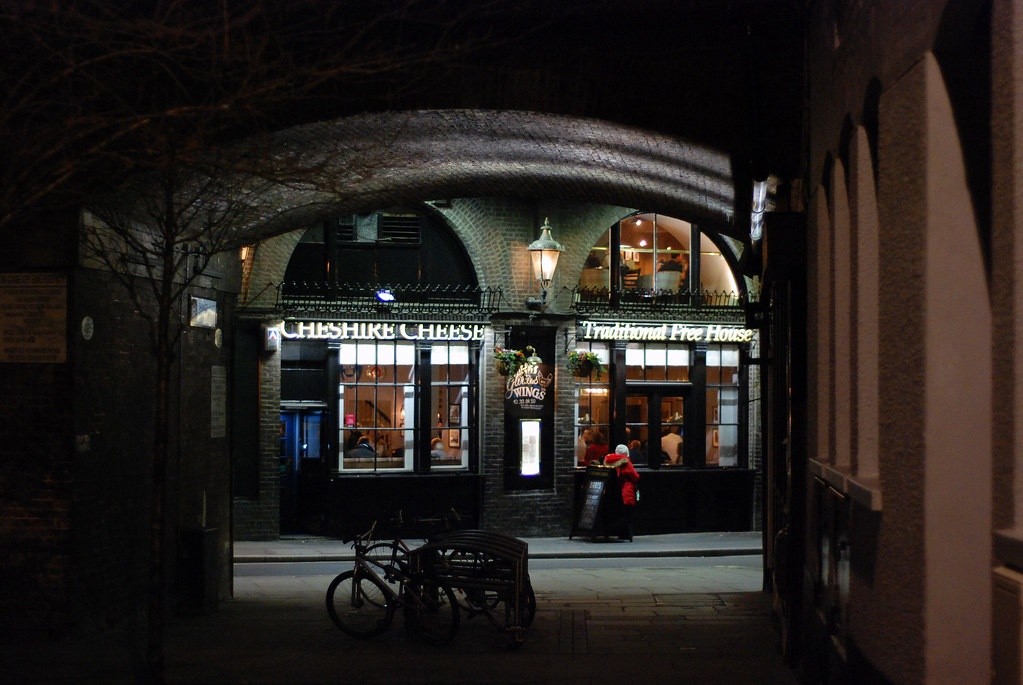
[623,267,641,289]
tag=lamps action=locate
[526,216,564,315]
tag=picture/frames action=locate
[712,428,720,448]
[452,403,461,427]
[448,429,460,448]
[661,400,673,423]
[712,405,719,424]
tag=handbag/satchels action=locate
[635,489,640,501]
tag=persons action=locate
[657,253,683,272]
[344,430,377,458]
[604,442,640,542]
[431,438,447,459]
[578,424,683,466]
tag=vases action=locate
[569,361,593,378]
[496,360,521,376]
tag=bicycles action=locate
[324,507,536,648]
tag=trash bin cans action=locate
[174,528,219,615]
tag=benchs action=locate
[343,457,461,468]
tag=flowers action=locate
[567,350,608,384]
[493,347,528,385]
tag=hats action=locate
[615,444,630,458]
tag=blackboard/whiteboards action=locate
[574,466,631,536]
